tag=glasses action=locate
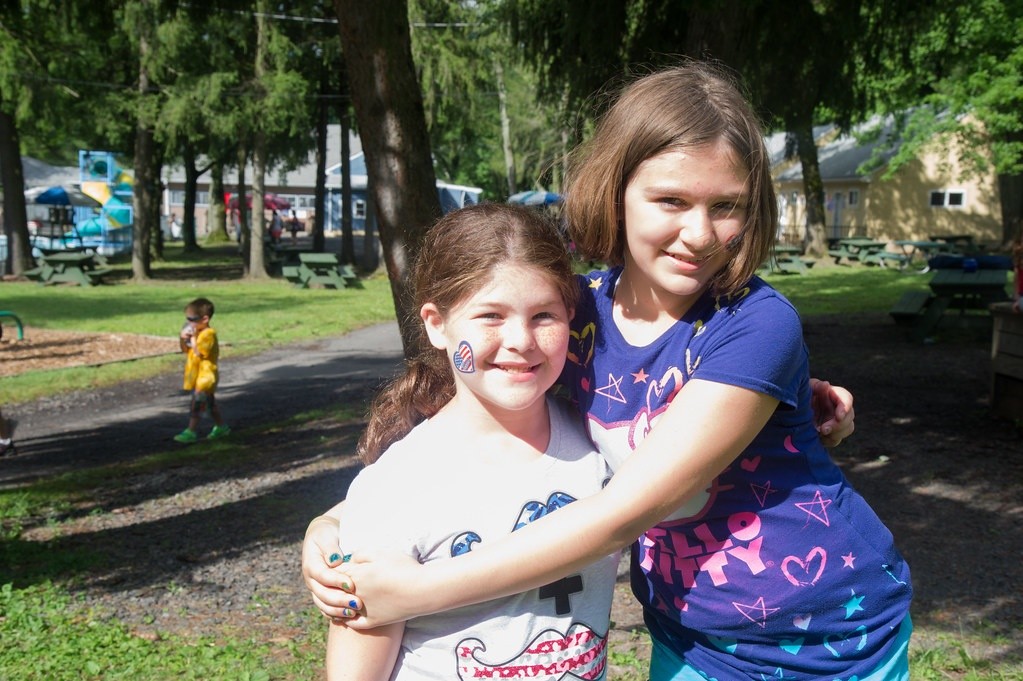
[185,315,204,322]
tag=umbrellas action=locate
[225,191,291,213]
[21,183,104,248]
[505,189,565,211]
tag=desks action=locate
[893,239,942,270]
[834,239,889,271]
[763,245,811,278]
[915,267,1016,346]
[34,251,99,287]
[294,252,347,291]
[928,235,980,259]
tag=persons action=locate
[301,63,914,681]
[1001,215,1023,300]
[316,199,860,681]
[269,209,284,243]
[174,298,231,442]
[289,210,300,245]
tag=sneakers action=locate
[206,423,232,441]
[174,429,198,445]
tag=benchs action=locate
[336,265,357,289]
[20,266,47,286]
[874,250,910,271]
[282,266,303,289]
[83,268,116,286]
[888,289,934,341]
[827,250,860,268]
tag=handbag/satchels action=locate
[926,253,1014,311]
